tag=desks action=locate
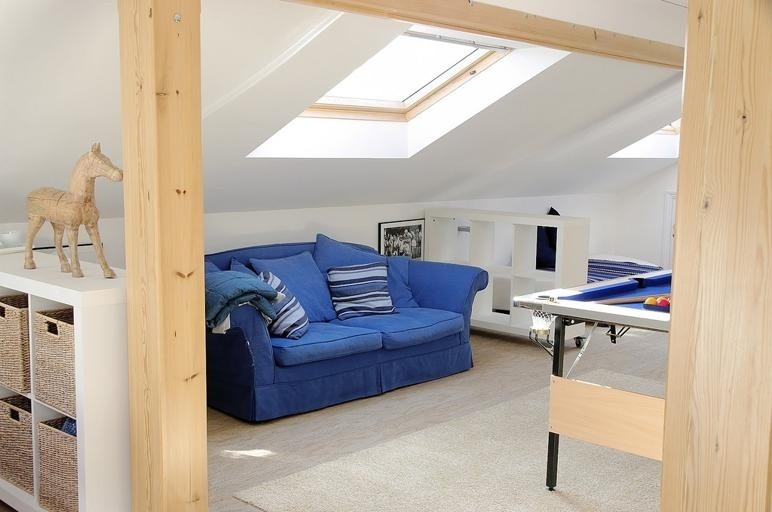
[511,269,672,493]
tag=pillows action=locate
[228,232,420,340]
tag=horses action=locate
[23,142,123,278]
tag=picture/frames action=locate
[377,218,425,262]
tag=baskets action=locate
[34,307,76,418]
[38,416,79,511]
[1,395,34,496]
[0,295,31,393]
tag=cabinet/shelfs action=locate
[421,207,590,343]
[0,250,128,512]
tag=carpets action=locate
[231,365,665,512]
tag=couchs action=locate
[205,240,491,422]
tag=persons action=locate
[384,225,422,259]
